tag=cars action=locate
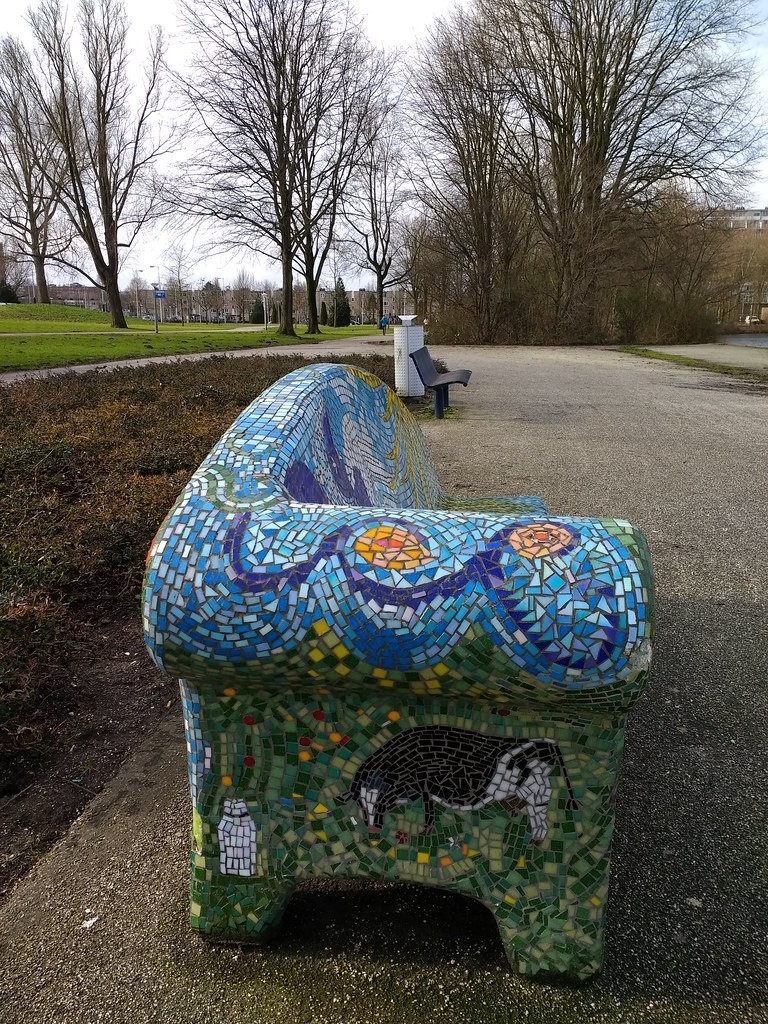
[350,320,358,325]
[132,316,142,318]
[158,318,168,322]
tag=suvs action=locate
[143,316,150,320]
[169,316,182,323]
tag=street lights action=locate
[135,270,143,318]
[218,278,224,290]
[332,273,349,327]
[261,293,268,330]
[150,266,163,323]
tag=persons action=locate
[380,315,387,335]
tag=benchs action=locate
[141,365,657,985]
[409,346,472,420]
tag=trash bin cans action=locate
[394,314,425,397]
